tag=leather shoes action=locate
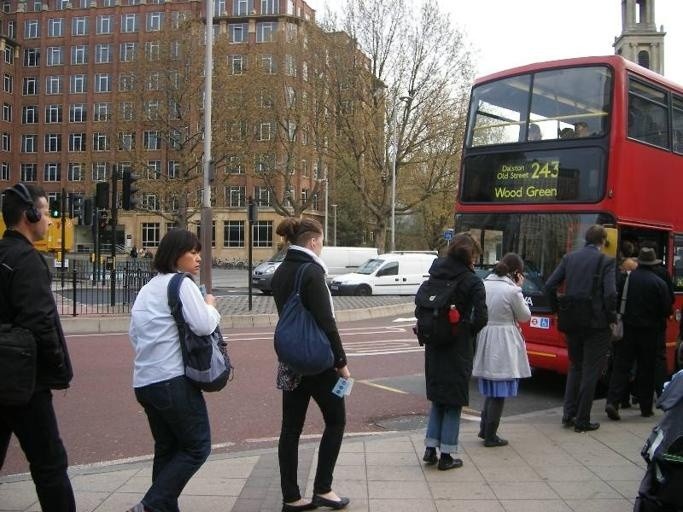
[437,457,463,471]
[561,415,574,427]
[574,418,600,433]
[476,431,484,439]
[484,435,509,447]
[421,449,436,465]
[311,494,351,509]
[281,499,318,512]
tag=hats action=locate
[632,246,663,265]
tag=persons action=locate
[575,122,589,138]
[0,181,77,512]
[616,238,675,408]
[470,252,532,448]
[527,124,542,141]
[543,225,619,433]
[633,370,683,512]
[130,245,153,258]
[270,217,350,512]
[127,230,222,512]
[605,247,669,421]
[413,232,488,471]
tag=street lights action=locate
[391,96,413,251]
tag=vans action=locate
[330,252,437,296]
[251,245,379,295]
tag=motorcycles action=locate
[209,256,256,270]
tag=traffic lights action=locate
[123,173,137,210]
[81,198,92,226]
[73,195,80,217]
[51,201,61,218]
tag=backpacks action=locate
[411,280,463,352]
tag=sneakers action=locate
[602,405,619,422]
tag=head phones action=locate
[4,183,41,223]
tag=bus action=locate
[455,55,683,395]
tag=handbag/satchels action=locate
[607,335,630,373]
[556,293,594,335]
[178,319,235,393]
[270,291,334,378]
